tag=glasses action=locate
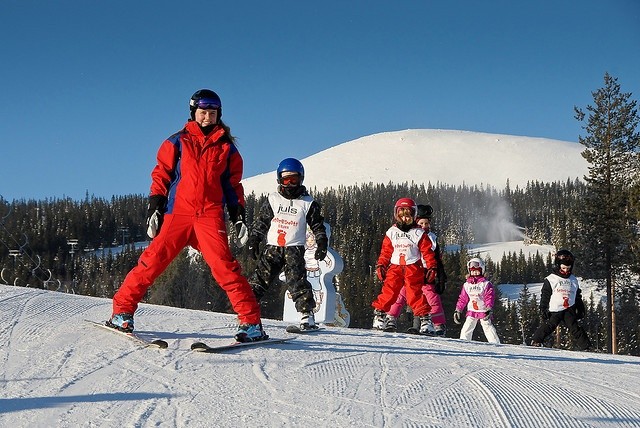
[556,254,572,261]
[199,98,218,110]
[469,261,480,269]
[276,173,302,186]
[418,205,433,216]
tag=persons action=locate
[385,205,448,335]
[246,158,328,329]
[452,258,501,344]
[105,89,268,344]
[532,249,590,351]
[371,199,435,337]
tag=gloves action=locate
[229,203,249,248]
[540,309,551,322]
[248,237,261,259]
[314,238,328,261]
[376,264,387,281]
[577,303,588,319]
[425,268,437,283]
[145,194,168,238]
[454,310,463,324]
[484,310,493,321]
[434,282,445,295]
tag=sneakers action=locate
[300,312,315,329]
[109,313,134,331]
[419,317,434,336]
[234,324,266,342]
[372,307,383,329]
[407,317,419,334]
[434,324,445,335]
[384,314,396,331]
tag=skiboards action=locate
[384,325,433,336]
[286,322,325,334]
[84,319,297,353]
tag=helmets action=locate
[190,89,221,113]
[277,158,304,180]
[555,250,575,274]
[466,258,485,275]
[394,199,418,222]
